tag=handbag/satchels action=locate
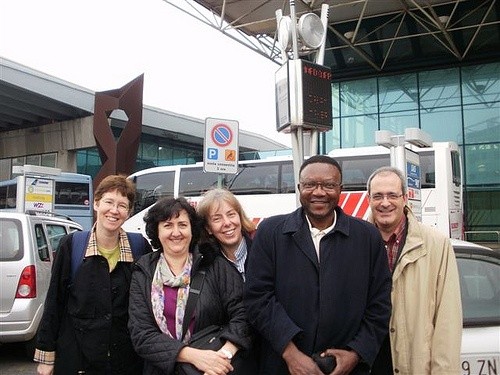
[179,325,244,375]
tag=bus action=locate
[0,163,95,232]
[116,137,466,242]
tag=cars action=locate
[449,239,500,375]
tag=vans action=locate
[0,208,83,363]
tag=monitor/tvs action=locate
[451,150,462,186]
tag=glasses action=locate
[303,181,344,191]
[372,193,405,201]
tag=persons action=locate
[243,156,392,375]
[33,175,154,375]
[365,167,464,375]
[197,189,257,375]
[127,196,252,375]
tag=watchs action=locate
[220,348,232,359]
